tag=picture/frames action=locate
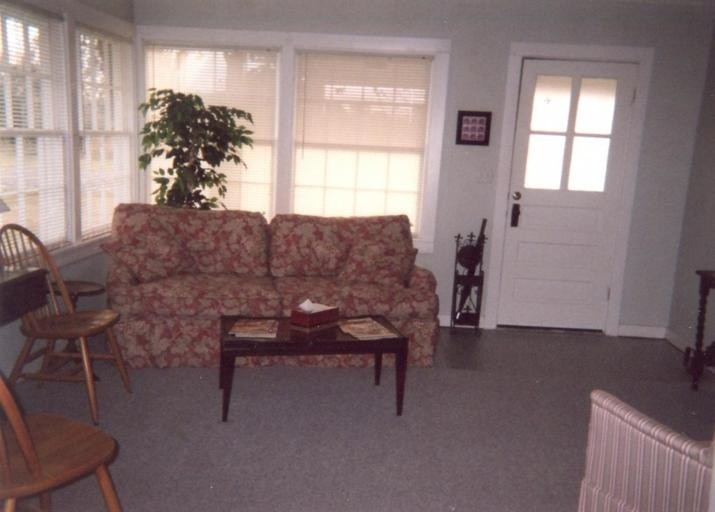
[454,110,492,146]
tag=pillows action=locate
[102,223,196,282]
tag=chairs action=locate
[0,375,123,512]
[1,223,132,426]
[579,389,713,512]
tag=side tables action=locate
[47,277,106,381]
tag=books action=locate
[338,317,397,341]
[228,315,280,338]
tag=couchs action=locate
[103,203,441,370]
